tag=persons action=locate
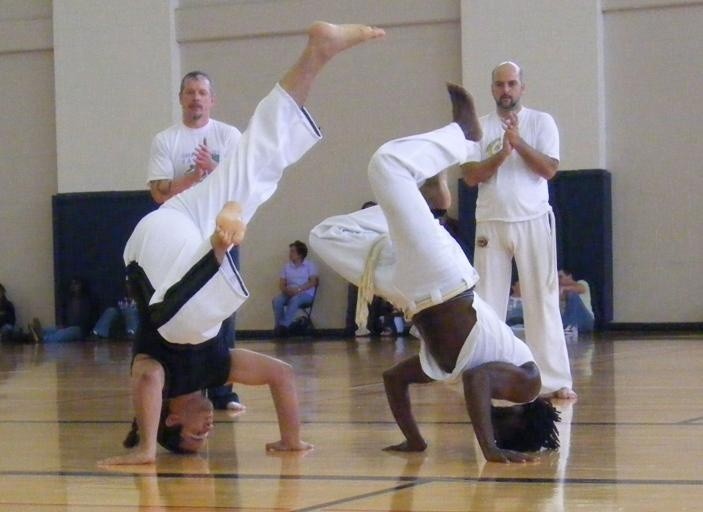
[377,300,406,339]
[460,60,579,399]
[308,80,559,463]
[96,22,385,466]
[28,277,92,343]
[506,267,596,334]
[145,70,247,410]
[1,287,16,342]
[93,278,141,341]
[272,241,317,341]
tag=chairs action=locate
[278,278,323,335]
